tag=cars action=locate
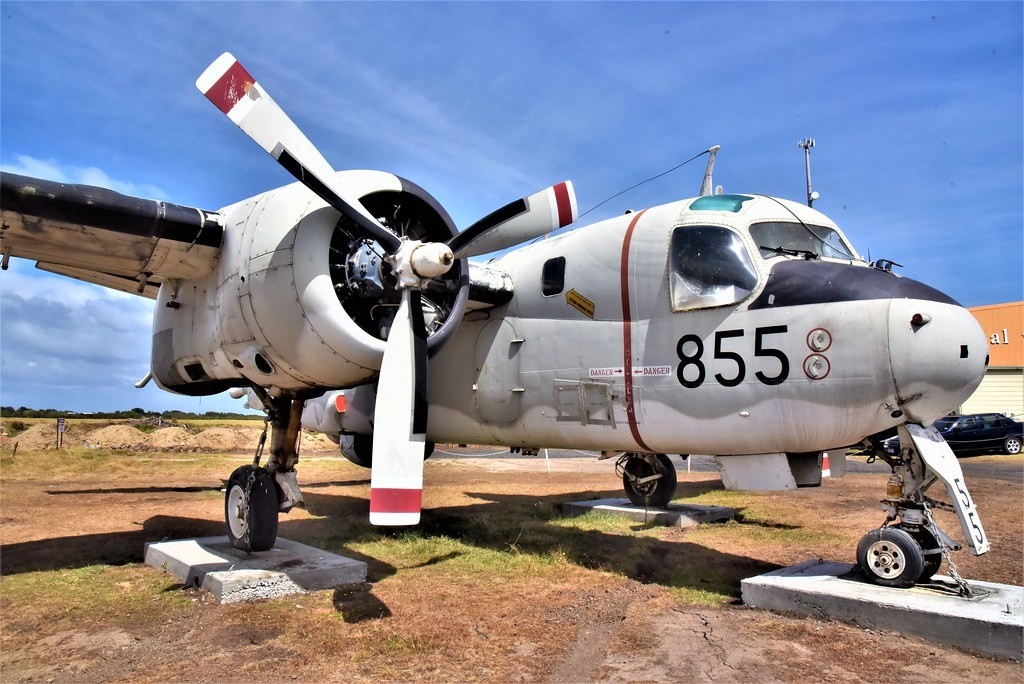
[883,412,1024,456]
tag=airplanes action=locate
[0,48,994,590]
